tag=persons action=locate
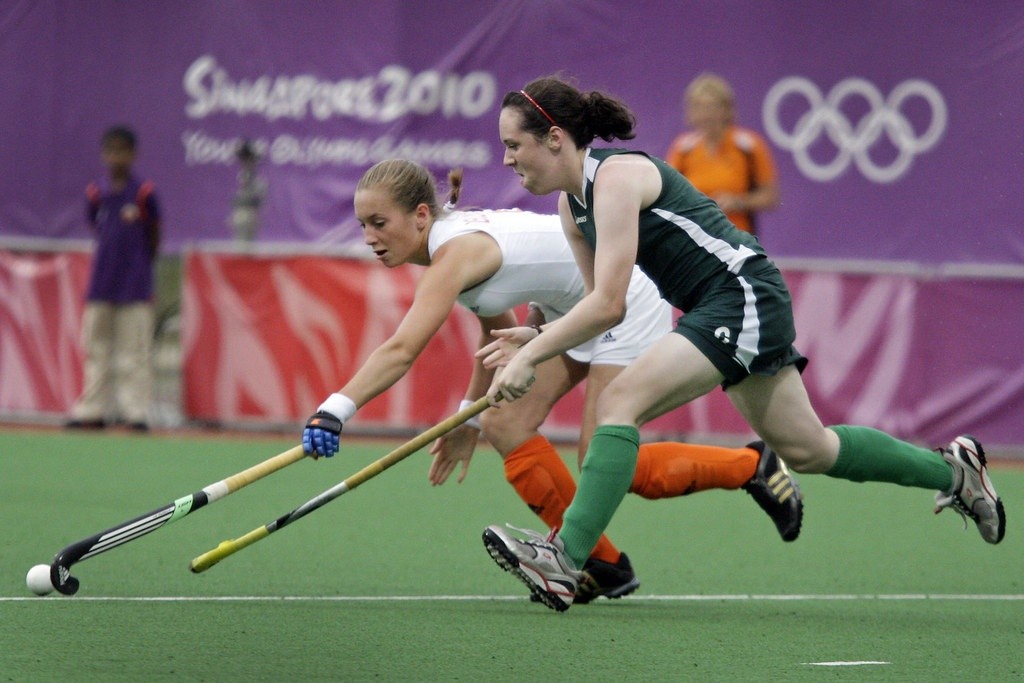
[300,157,805,605]
[468,76,1007,612]
[63,126,164,431]
[664,72,781,239]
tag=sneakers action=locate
[530,552,640,604]
[742,442,804,543]
[483,524,586,612]
[934,434,1006,546]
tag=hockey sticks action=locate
[189,374,538,574]
[52,441,314,596]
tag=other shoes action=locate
[66,419,105,429]
[128,422,151,434]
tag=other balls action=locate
[26,563,54,594]
